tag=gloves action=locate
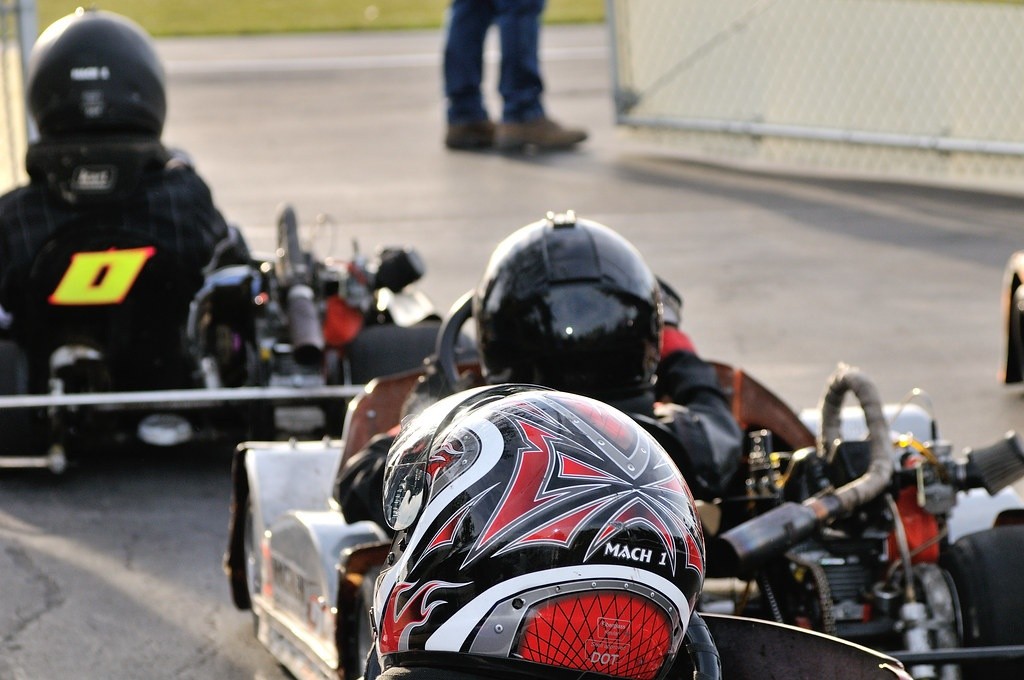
[655,272,682,329]
[400,354,472,425]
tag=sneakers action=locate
[445,121,499,148]
[492,115,590,149]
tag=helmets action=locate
[473,212,662,406]
[370,378,705,675]
[26,7,166,140]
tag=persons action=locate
[328,206,747,544]
[0,1,269,478]
[437,0,589,157]
[345,378,714,680]
